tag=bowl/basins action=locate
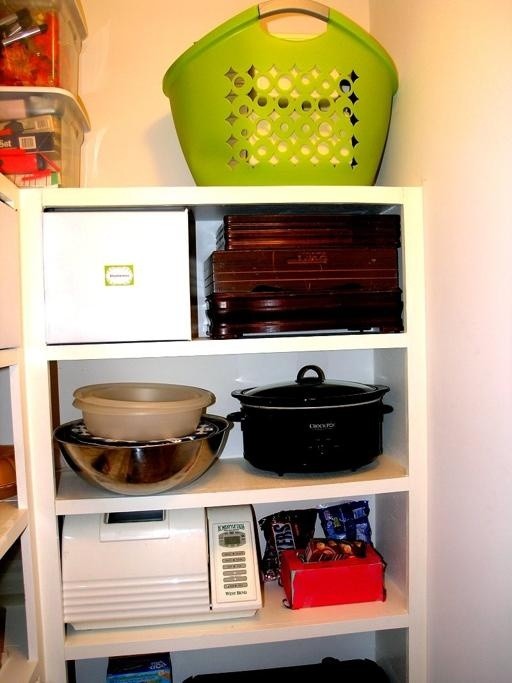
[51,415,234,496]
[72,384,212,438]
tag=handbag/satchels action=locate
[163,0,399,186]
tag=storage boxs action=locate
[1,1,89,187]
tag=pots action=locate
[226,365,394,478]
